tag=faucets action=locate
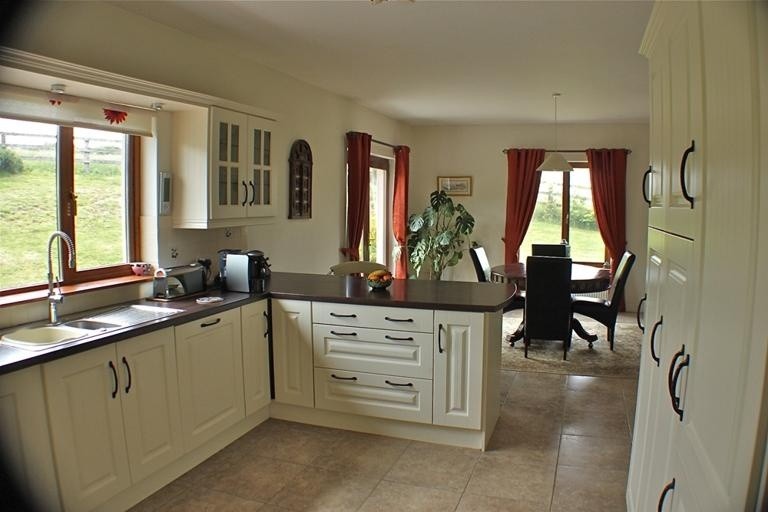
[46,230,76,324]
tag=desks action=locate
[490,264,611,351]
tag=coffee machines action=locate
[225,249,271,293]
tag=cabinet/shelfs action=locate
[169,106,287,232]
[622,0,768,511]
[1,364,62,511]
[175,308,246,468]
[310,297,433,444]
[240,299,276,435]
[42,326,181,512]
[431,306,503,451]
[267,298,315,425]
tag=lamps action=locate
[535,91,572,172]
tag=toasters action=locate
[153,263,207,299]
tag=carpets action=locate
[502,316,642,378]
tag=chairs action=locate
[467,243,527,312]
[532,243,566,257]
[325,261,386,278]
[569,251,636,342]
[523,256,572,361]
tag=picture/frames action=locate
[435,176,472,195]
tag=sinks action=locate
[64,320,122,331]
[0,327,88,351]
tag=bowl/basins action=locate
[368,281,391,288]
[131,263,151,275]
